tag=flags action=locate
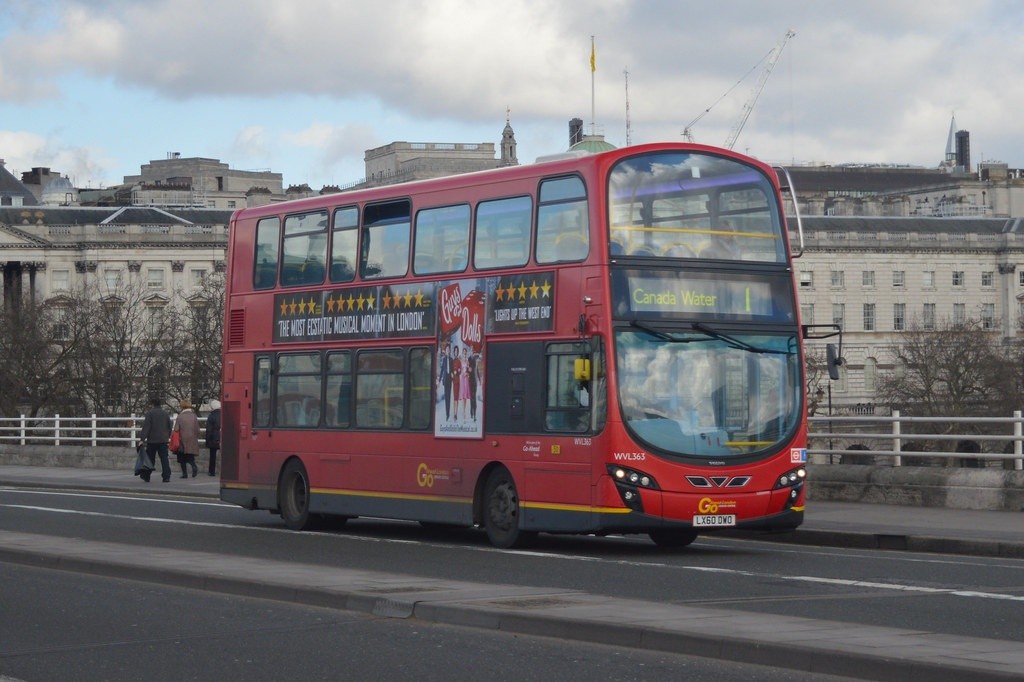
[590,39,596,74]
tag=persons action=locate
[138,399,172,484]
[174,399,200,480]
[439,344,477,421]
[205,400,220,477]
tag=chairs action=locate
[256,232,696,286]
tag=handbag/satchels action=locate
[169,430,179,453]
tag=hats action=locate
[210,399,221,409]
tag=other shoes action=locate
[140,474,150,482]
[208,472,215,476]
[162,478,170,482]
[192,466,198,477]
[180,473,188,478]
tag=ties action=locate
[447,357,450,374]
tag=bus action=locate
[217,141,843,554]
